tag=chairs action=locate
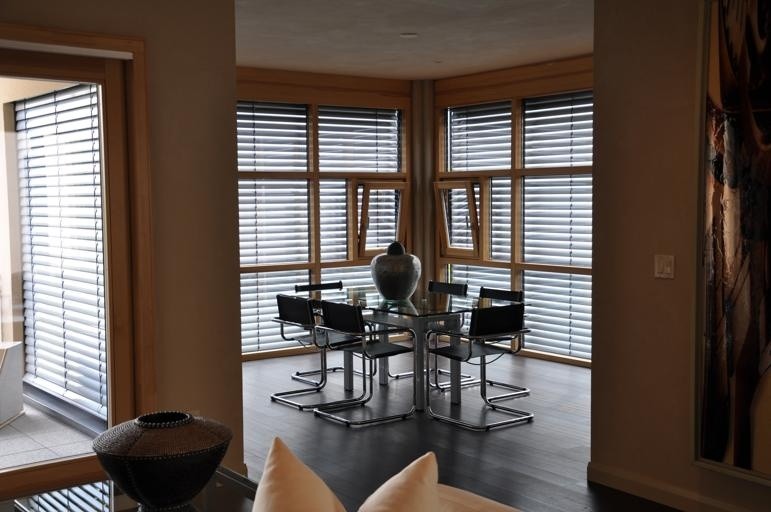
[269,280,533,432]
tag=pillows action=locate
[358,451,440,511]
[253,436,346,512]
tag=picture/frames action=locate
[689,0,771,488]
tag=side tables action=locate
[0,451,258,512]
